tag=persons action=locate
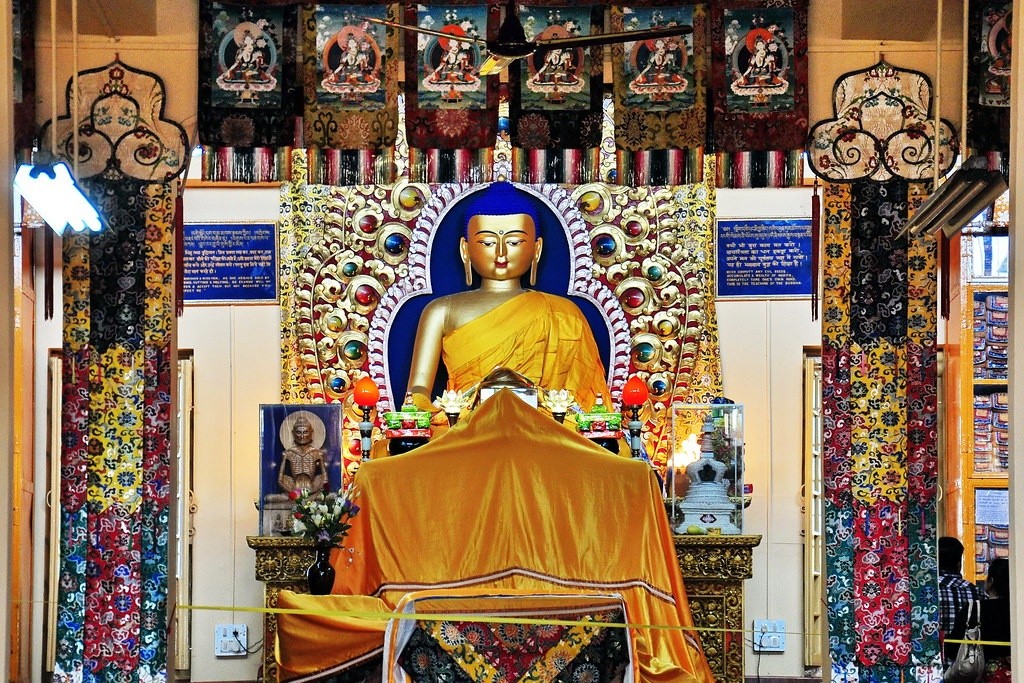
[945,558,1011,665]
[284,514,293,530]
[271,514,283,533]
[401,184,631,458]
[278,416,328,500]
[938,536,989,666]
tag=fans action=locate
[356,0,697,77]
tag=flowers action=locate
[289,483,364,568]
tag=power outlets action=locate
[753,620,785,652]
[214,623,248,656]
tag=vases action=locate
[307,547,336,595]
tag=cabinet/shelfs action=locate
[963,284,1009,584]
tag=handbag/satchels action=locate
[943,582,985,683]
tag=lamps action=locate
[436,389,472,428]
[541,388,573,424]
[354,376,380,462]
[11,0,115,237]
[622,376,649,460]
[895,0,1009,240]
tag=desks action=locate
[274,585,385,683]
[247,536,759,683]
